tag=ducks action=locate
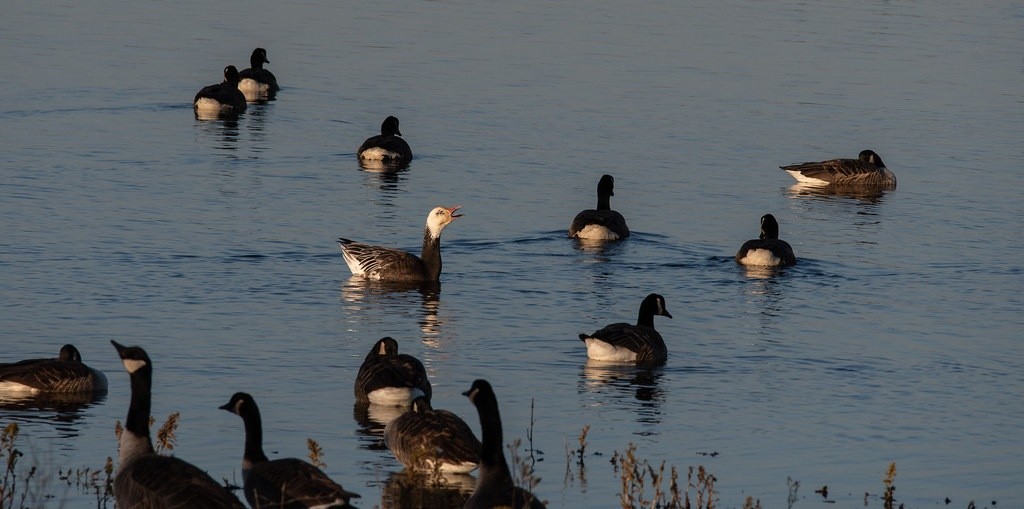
[736,214,796,268]
[1,343,101,394]
[336,205,465,284]
[193,65,247,112]
[569,173,630,240]
[383,398,481,472]
[356,115,413,166]
[238,48,279,92]
[354,336,432,405]
[218,391,362,509]
[580,293,673,363]
[461,378,548,509]
[109,337,247,508]
[775,149,896,186]
